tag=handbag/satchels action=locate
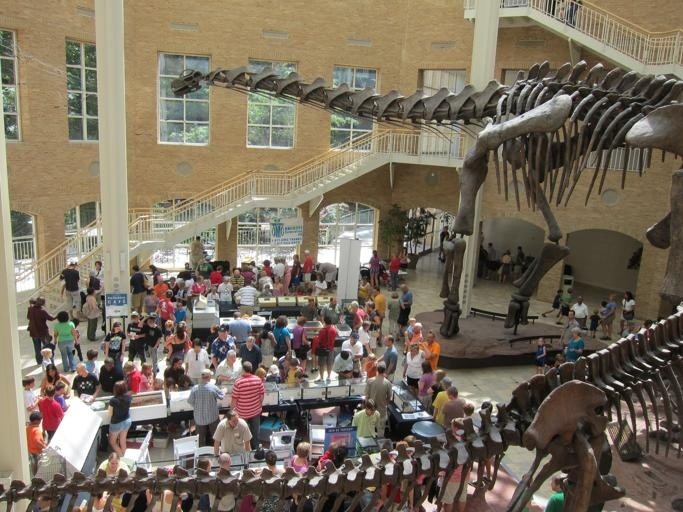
[316,344,331,358]
[372,320,381,338]
[297,343,309,360]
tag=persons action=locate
[546,0,584,29]
[521,473,568,512]
[496,246,536,284]
[535,288,682,376]
[22,224,496,512]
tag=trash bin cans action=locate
[562,275,575,301]
[107,307,129,337]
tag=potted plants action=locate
[377,203,436,269]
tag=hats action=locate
[218,323,231,333]
[199,368,215,377]
[128,310,139,318]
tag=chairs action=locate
[197,446,221,465]
[309,424,328,461]
[173,434,200,468]
[269,429,297,455]
[119,430,152,469]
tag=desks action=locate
[263,306,322,320]
[290,334,352,348]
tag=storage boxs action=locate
[168,372,426,414]
[297,295,315,307]
[257,296,276,308]
[317,296,332,311]
[277,295,297,306]
[335,323,352,337]
